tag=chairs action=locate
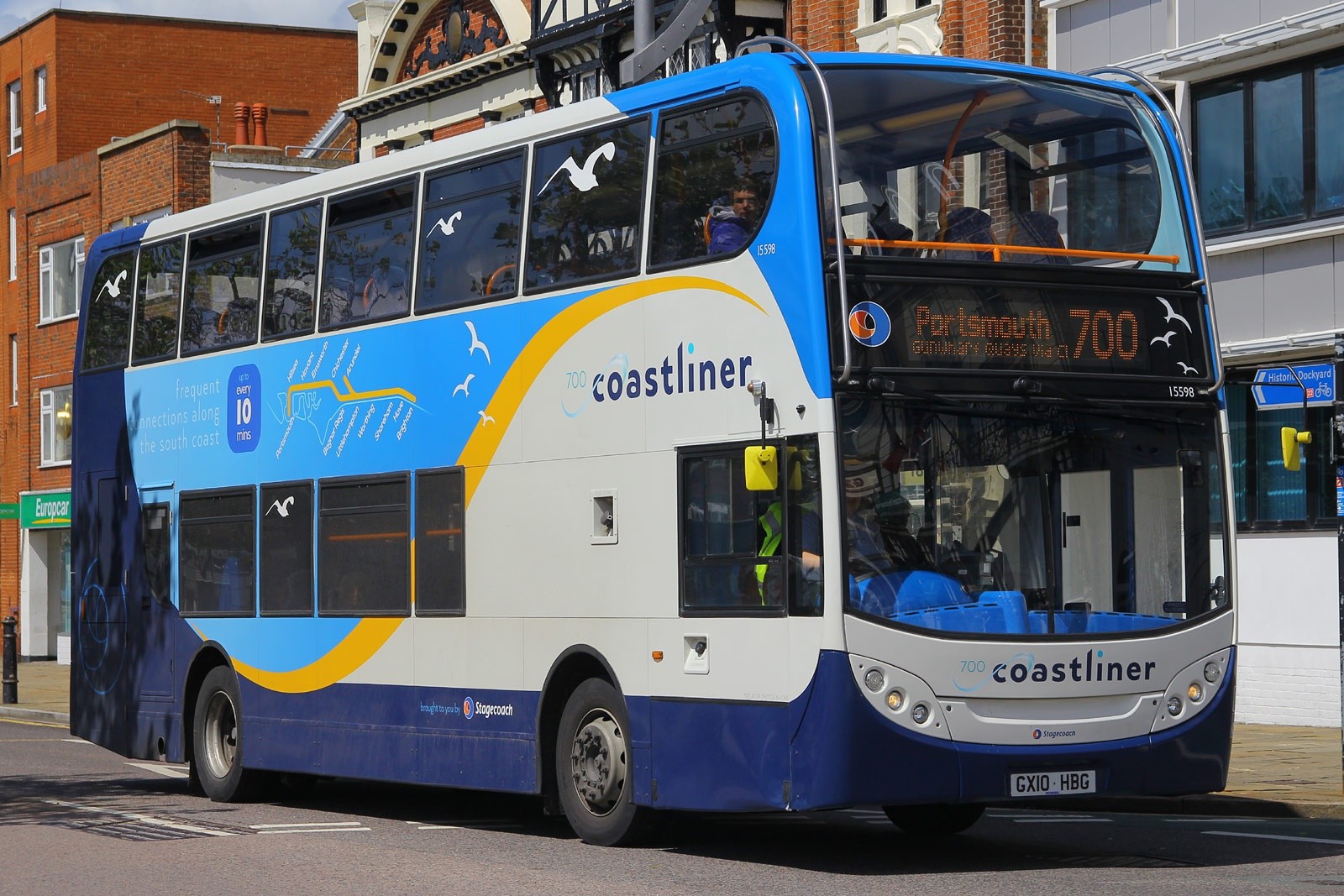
[130,194,1076,359]
[182,550,463,612]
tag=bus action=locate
[66,33,1315,852]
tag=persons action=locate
[707,178,764,254]
[801,477,937,581]
